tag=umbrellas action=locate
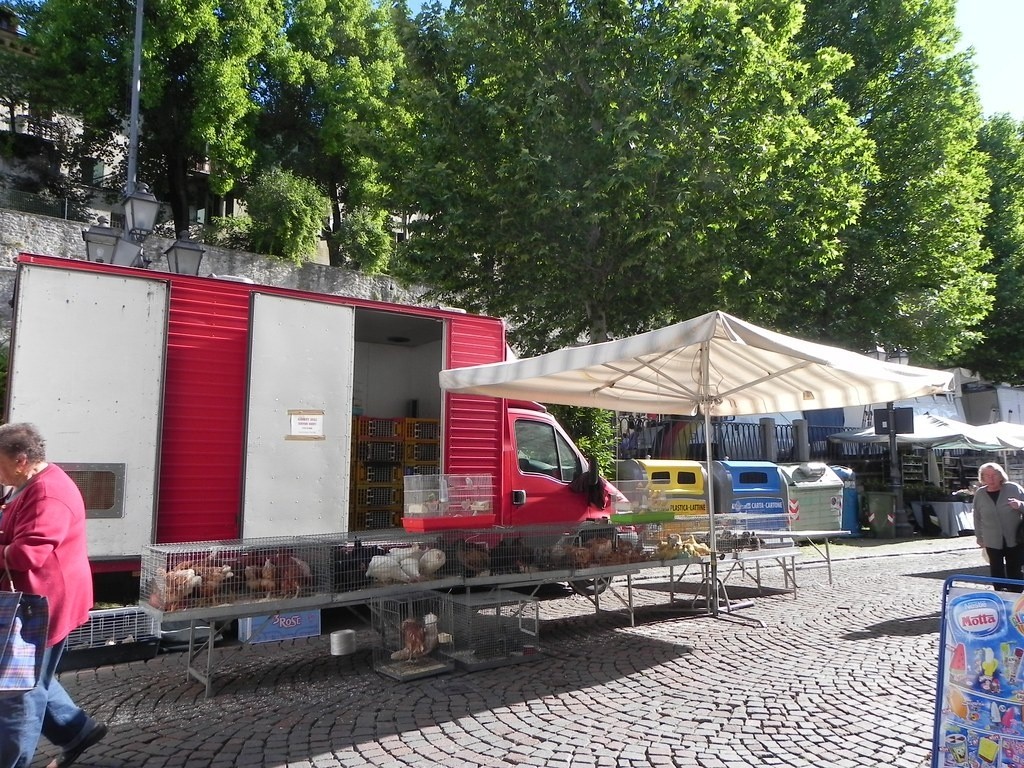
[826,411,1024,482]
[439,311,955,614]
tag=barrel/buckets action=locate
[331,629,357,656]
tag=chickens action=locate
[450,532,651,572]
[650,532,711,560]
[156,554,312,608]
[71,633,135,650]
[391,618,439,660]
[338,536,447,586]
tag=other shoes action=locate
[47,724,108,768]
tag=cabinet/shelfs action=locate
[903,454,933,487]
[837,449,891,486]
[943,456,1000,490]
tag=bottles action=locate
[439,480,448,502]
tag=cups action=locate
[523,645,535,656]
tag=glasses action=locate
[982,475,1000,479]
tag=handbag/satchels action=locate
[0,546,48,690]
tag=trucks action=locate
[2,250,640,642]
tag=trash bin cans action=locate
[867,492,898,539]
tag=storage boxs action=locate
[237,608,321,643]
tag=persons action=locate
[0,423,108,768]
[972,463,1024,594]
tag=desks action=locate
[907,500,974,537]
